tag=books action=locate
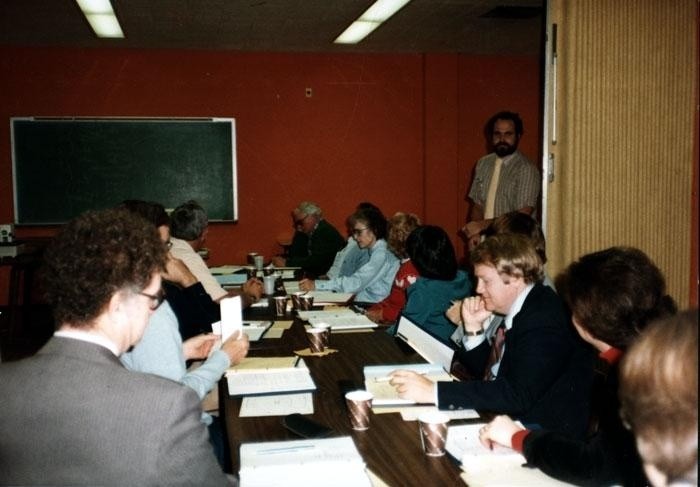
[363,363,456,408]
[225,357,317,397]
[297,308,378,330]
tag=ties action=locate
[481,158,502,243]
[485,327,506,381]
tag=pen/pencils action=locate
[488,440,493,450]
[242,323,261,326]
[374,372,428,383]
[302,270,306,279]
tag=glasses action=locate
[352,228,368,232]
[140,290,163,309]
[296,215,308,224]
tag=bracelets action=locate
[465,328,484,336]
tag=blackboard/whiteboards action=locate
[9,116,239,227]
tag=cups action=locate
[273,296,290,317]
[417,412,453,459]
[264,277,277,295]
[300,295,314,311]
[344,389,375,433]
[263,267,275,276]
[291,292,306,309]
[247,253,264,271]
[312,322,332,350]
[305,328,327,355]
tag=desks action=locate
[209,264,578,487]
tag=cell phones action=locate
[279,413,334,439]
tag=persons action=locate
[272,200,555,366]
[617,310,699,486]
[1,207,240,485]
[463,112,542,250]
[118,201,249,427]
[479,247,677,487]
[386,233,597,432]
